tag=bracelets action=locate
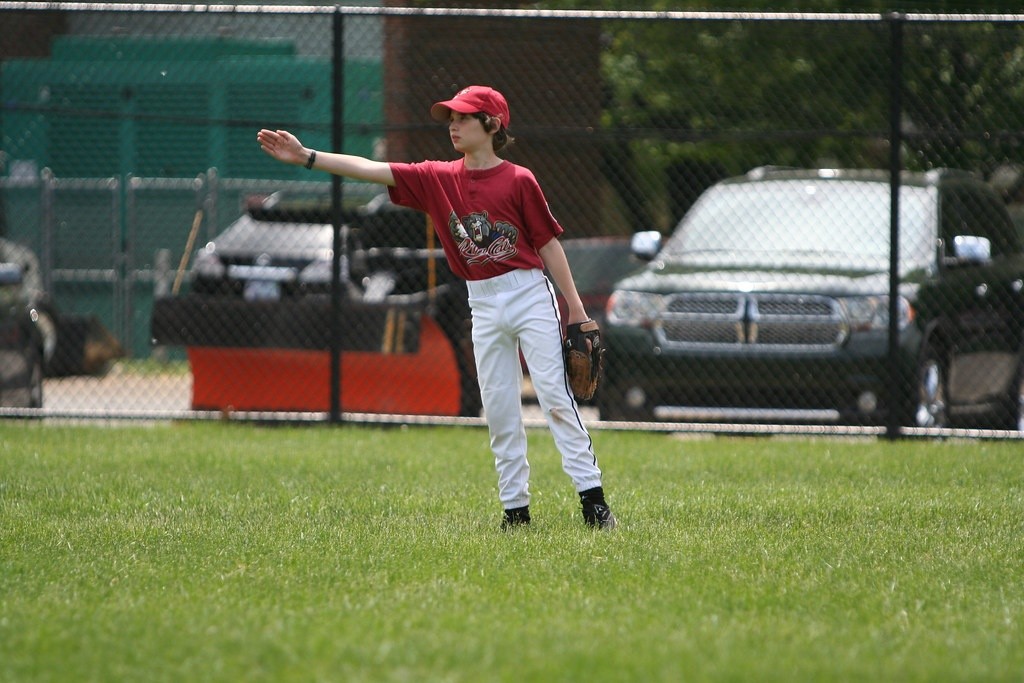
[305,150,316,170]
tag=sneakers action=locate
[581,502,614,528]
[498,505,530,528]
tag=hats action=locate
[431,86,510,128]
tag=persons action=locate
[258,86,614,531]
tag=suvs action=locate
[189,189,482,417]
[601,163,1022,430]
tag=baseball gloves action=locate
[563,318,607,401]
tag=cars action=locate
[0,241,51,407]
